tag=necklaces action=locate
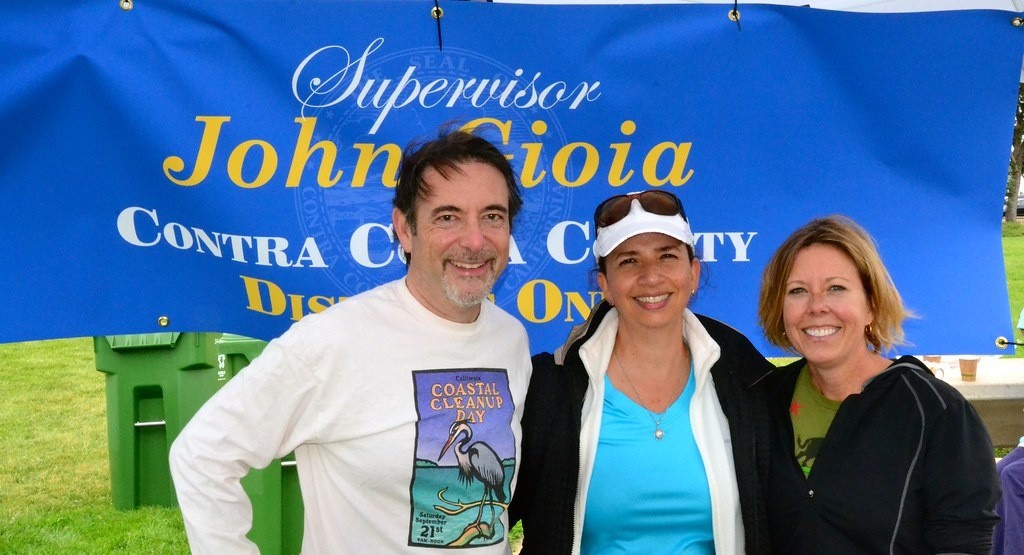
[614,346,688,439]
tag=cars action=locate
[1002,147,1024,217]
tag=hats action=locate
[593,191,695,268]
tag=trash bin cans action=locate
[215,334,305,555]
[93,331,228,511]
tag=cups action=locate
[959,357,979,382]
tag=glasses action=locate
[594,190,687,240]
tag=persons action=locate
[168,119,533,555]
[508,192,777,555]
[763,216,1024,555]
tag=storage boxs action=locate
[941,356,1024,447]
